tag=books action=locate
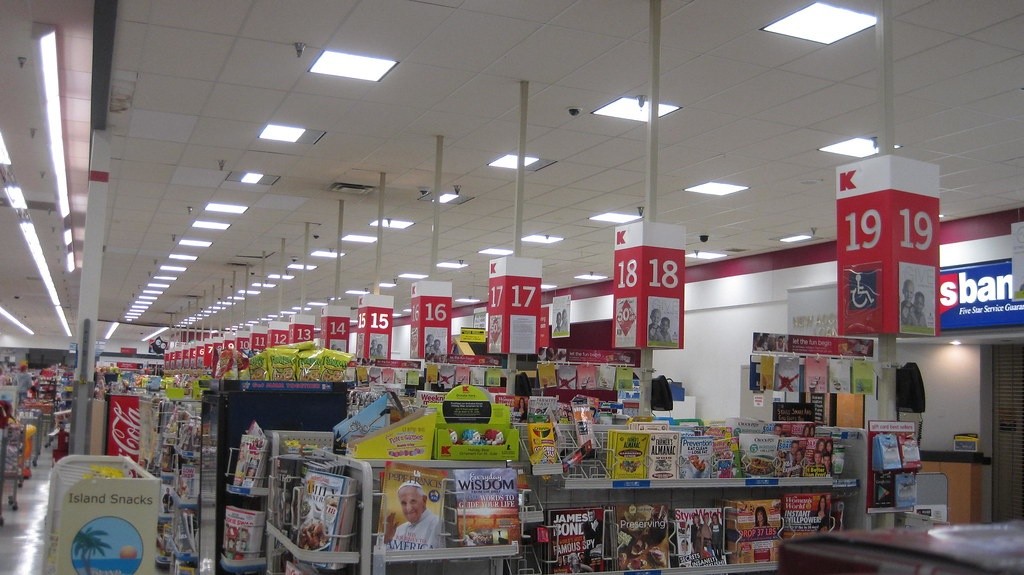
[378,460,519,551]
[225,420,357,575]
[543,420,834,574]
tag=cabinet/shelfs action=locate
[155,397,916,575]
[37,379,61,400]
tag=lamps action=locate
[31,21,70,219]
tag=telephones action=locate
[875,362,926,414]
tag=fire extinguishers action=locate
[44,420,70,469]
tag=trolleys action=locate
[0,399,61,511]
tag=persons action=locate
[371,340,383,358]
[825,440,833,453]
[516,399,528,423]
[554,309,568,332]
[813,451,827,476]
[710,512,722,561]
[245,467,256,480]
[787,448,805,477]
[582,509,603,553]
[774,424,782,437]
[691,514,702,552]
[701,513,712,557]
[382,480,443,549]
[809,425,815,436]
[426,335,441,355]
[802,425,810,437]
[753,506,771,562]
[778,440,799,467]
[824,452,832,477]
[811,496,830,532]
[815,439,825,451]
[902,280,927,328]
[649,309,672,343]
[0,364,32,400]
